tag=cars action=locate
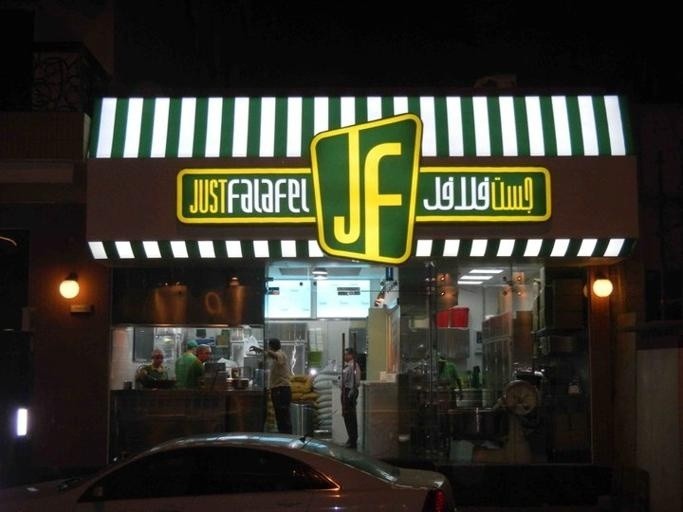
[0,431,456,512]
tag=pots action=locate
[451,387,482,409]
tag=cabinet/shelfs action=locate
[360,381,398,459]
[479,310,533,406]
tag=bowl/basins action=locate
[232,379,248,390]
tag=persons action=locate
[471,365,481,389]
[437,356,465,405]
[174,340,198,387]
[216,353,239,378]
[187,344,212,390]
[248,338,292,434]
[340,348,362,448]
[135,349,168,389]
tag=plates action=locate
[152,380,180,389]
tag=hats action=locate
[187,340,198,348]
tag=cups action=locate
[124,381,132,390]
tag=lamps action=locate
[57,272,80,298]
[591,268,613,298]
